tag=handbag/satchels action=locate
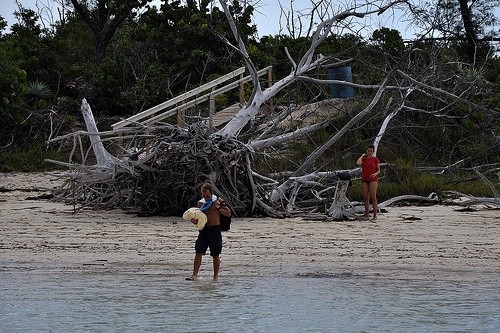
[214,194,232,231]
[336,172,352,186]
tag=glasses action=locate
[202,193,211,198]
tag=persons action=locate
[356,145,381,221]
[184,183,232,284]
